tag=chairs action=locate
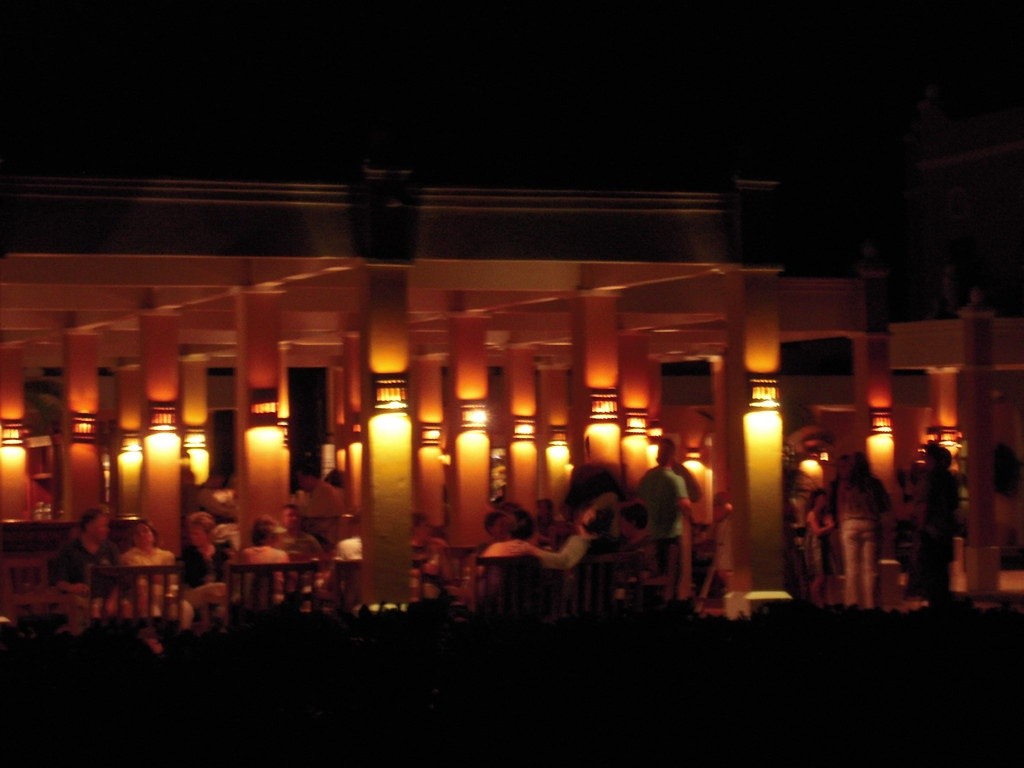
[227,560,322,609]
[565,550,643,614]
[621,534,683,605]
[89,563,187,629]
[473,551,542,614]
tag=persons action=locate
[50,457,346,632]
[410,437,723,615]
[782,442,1024,611]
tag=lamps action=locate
[512,419,536,439]
[120,432,144,452]
[183,429,207,449]
[749,378,781,408]
[249,400,277,424]
[547,429,568,446]
[872,411,893,433]
[590,392,618,419]
[625,412,647,434]
[461,403,487,428]
[148,406,178,432]
[421,426,441,445]
[374,379,408,409]
[1,423,24,445]
[70,415,98,440]
[939,432,956,447]
